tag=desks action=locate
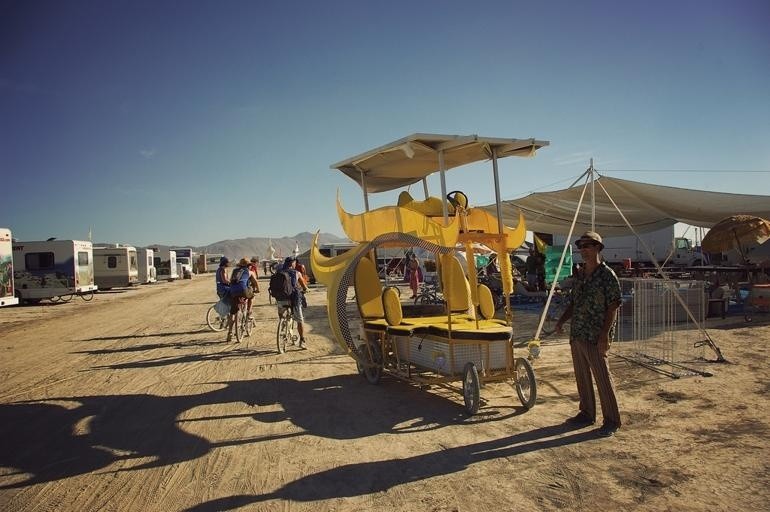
[629,265,770,285]
[547,287,562,296]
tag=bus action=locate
[1,228,193,308]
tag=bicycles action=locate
[414,277,504,310]
[206,283,260,343]
[277,290,310,354]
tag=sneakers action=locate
[247,310,253,316]
[566,412,595,427]
[596,422,622,437]
[227,332,233,342]
[299,341,308,350]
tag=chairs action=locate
[513,282,547,302]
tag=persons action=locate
[226,258,261,342]
[215,255,231,300]
[247,256,259,280]
[293,258,306,278]
[276,256,308,350]
[553,230,624,439]
[408,254,419,299]
[486,252,499,276]
[524,248,537,284]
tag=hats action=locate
[236,256,254,267]
[575,230,605,251]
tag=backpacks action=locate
[268,269,296,300]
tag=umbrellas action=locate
[699,213,770,284]
